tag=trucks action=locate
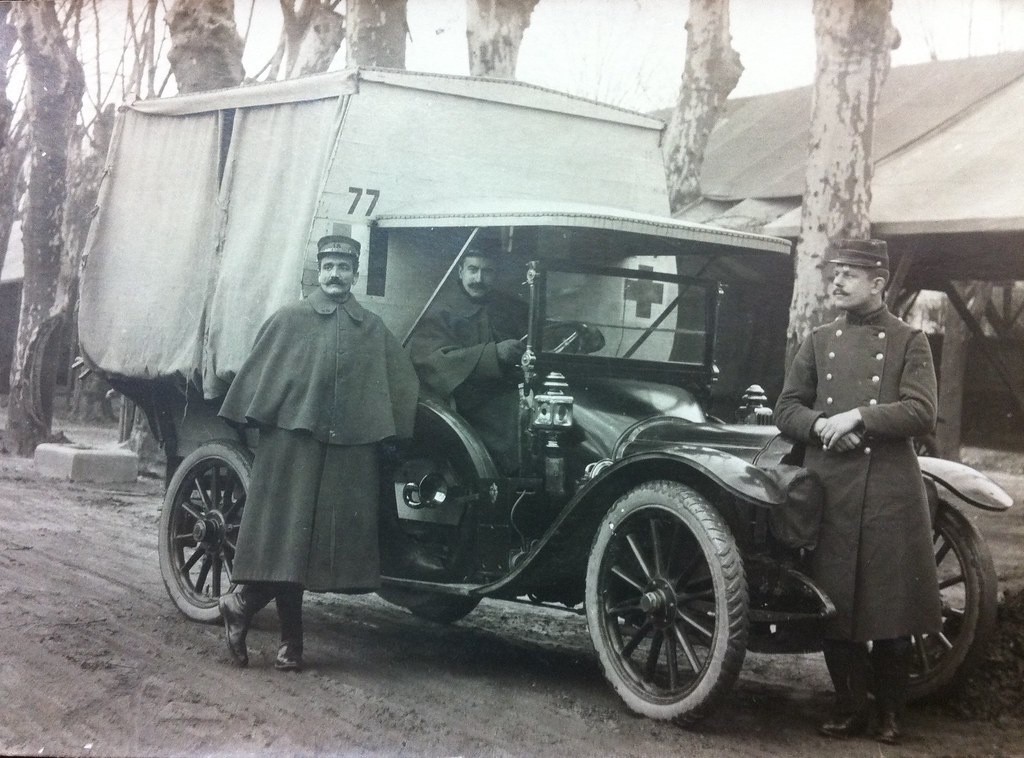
[76,68,1014,729]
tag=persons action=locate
[216,234,419,671]
[774,239,942,745]
[407,238,605,473]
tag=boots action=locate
[815,636,870,739]
[275,582,305,669]
[870,634,913,744]
[218,581,281,666]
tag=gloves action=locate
[496,339,528,367]
[579,321,601,350]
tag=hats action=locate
[460,232,504,266]
[317,235,361,261]
[829,238,890,271]
